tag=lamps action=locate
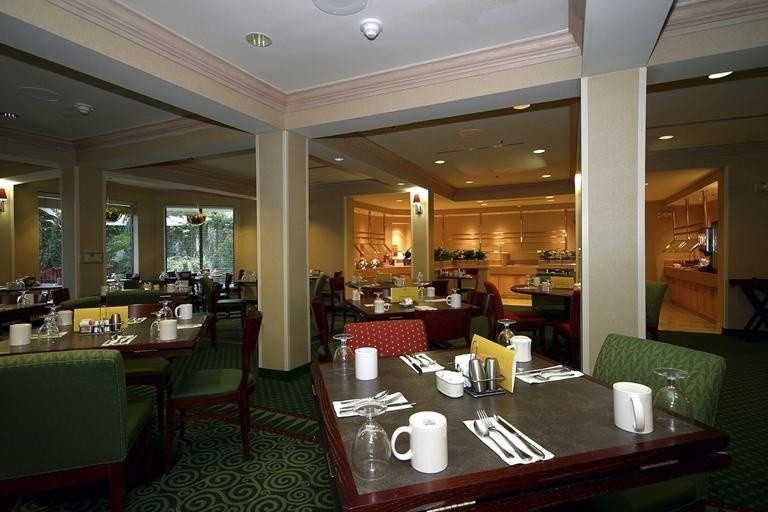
[412,193,423,214]
[0,189,8,212]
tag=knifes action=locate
[339,388,417,415]
[492,413,546,459]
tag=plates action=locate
[399,302,412,307]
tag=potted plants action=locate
[187,211,206,226]
[106,206,123,222]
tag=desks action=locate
[729,277,767,342]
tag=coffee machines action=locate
[696,227,717,272]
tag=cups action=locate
[541,282,549,293]
[355,347,379,380]
[506,336,533,363]
[332,334,356,377]
[158,320,179,341]
[612,381,655,436]
[426,287,436,298]
[530,277,540,288]
[446,294,462,309]
[175,277,185,292]
[56,310,74,326]
[352,291,365,301]
[389,409,450,475]
[174,304,194,319]
[374,299,390,314]
[8,323,33,346]
[393,271,425,298]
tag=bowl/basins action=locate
[436,369,464,399]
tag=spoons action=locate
[473,418,514,460]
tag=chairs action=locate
[309,270,729,512]
[1,269,262,512]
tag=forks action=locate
[516,364,576,381]
[476,409,533,461]
[403,351,442,372]
[105,334,132,344]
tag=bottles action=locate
[110,313,121,331]
[470,359,487,393]
[484,357,498,392]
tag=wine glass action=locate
[496,319,518,348]
[149,310,168,340]
[37,305,65,336]
[16,290,30,305]
[158,295,173,321]
[652,367,694,433]
[351,399,395,483]
